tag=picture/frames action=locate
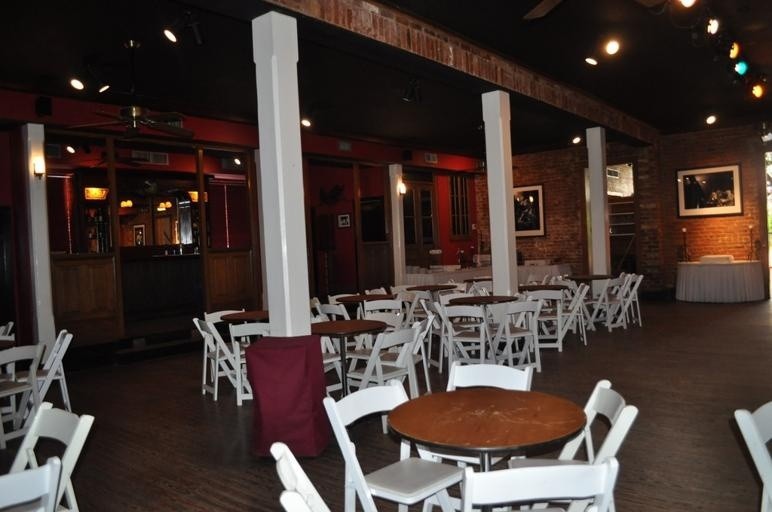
[337,214,351,228]
[514,184,546,236]
[676,164,742,216]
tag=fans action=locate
[82,151,150,167]
[64,39,194,138]
[463,160,518,176]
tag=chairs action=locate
[0,457,60,512]
[465,457,619,512]
[0,322,73,450]
[322,379,465,512]
[424,361,533,512]
[0,401,94,512]
[504,380,638,512]
[734,401,772,512]
[193,310,270,407]
[270,441,331,512]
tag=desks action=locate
[388,389,587,512]
[676,261,765,303]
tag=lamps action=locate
[64,63,111,94]
[635,0,765,99]
[403,79,421,104]
[160,10,190,44]
